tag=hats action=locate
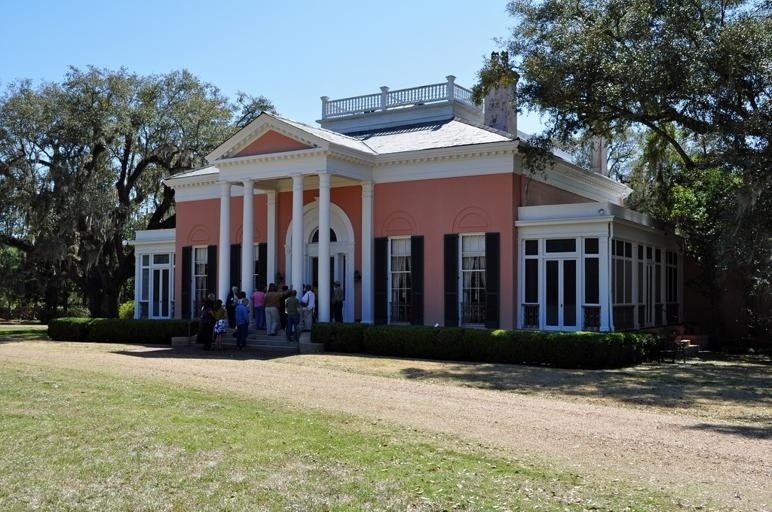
[335,281,340,285]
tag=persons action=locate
[193,279,318,351]
[331,280,344,323]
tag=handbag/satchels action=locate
[300,301,308,307]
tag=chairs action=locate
[660,342,685,364]
